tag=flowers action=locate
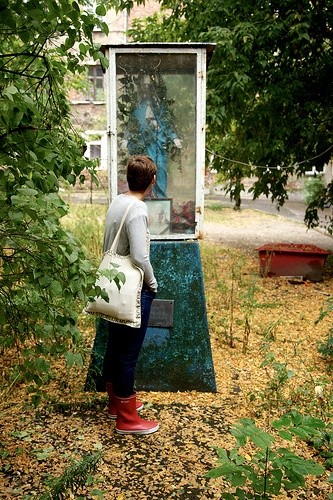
[171,200,195,231]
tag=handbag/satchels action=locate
[84,249,145,329]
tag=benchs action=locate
[256,243,330,278]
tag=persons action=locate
[118,74,183,198]
[103,154,160,434]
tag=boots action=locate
[106,382,143,419]
[114,392,160,435]
